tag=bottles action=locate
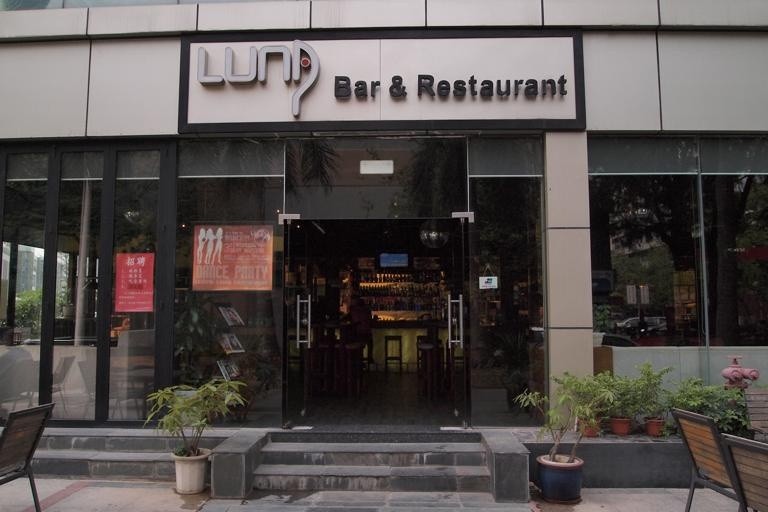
[358,281,439,311]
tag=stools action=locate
[384,334,403,376]
[416,333,447,403]
[335,341,369,403]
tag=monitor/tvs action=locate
[374,250,413,268]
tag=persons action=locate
[338,293,374,364]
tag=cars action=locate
[613,316,727,347]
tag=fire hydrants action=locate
[721,353,760,404]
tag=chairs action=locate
[1,402,55,511]
[1,355,156,421]
[670,390,767,512]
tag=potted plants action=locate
[513,371,615,505]
[59,284,76,317]
[141,373,248,496]
[549,363,676,436]
